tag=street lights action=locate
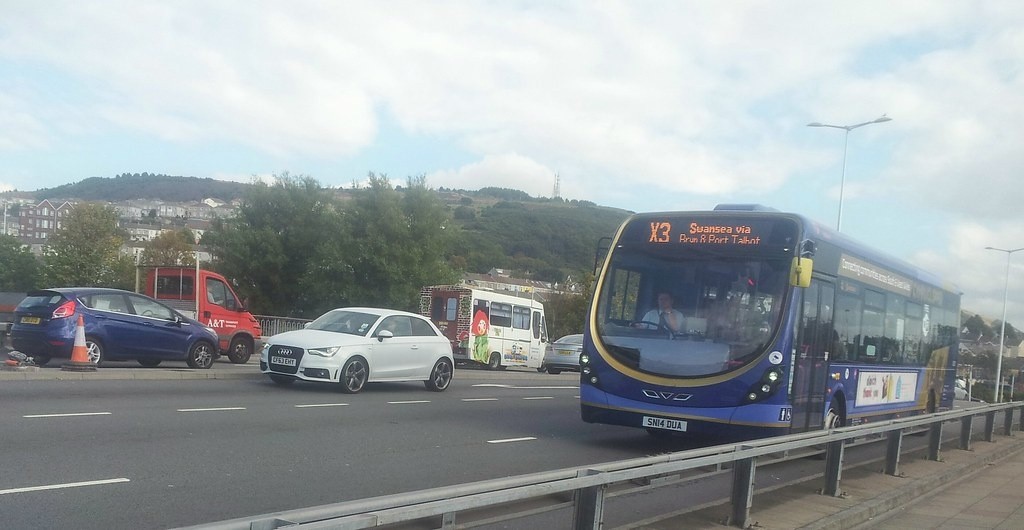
[982,246,1024,405]
[805,114,896,233]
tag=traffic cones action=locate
[62,314,100,373]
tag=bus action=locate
[578,203,963,453]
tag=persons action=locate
[207,285,224,305]
[628,288,684,337]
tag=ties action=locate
[659,314,665,330]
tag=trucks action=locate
[419,285,551,371]
[1,260,265,366]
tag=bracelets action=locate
[667,311,672,314]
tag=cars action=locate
[10,286,223,370]
[260,307,455,393]
[543,333,584,375]
[952,381,969,400]
